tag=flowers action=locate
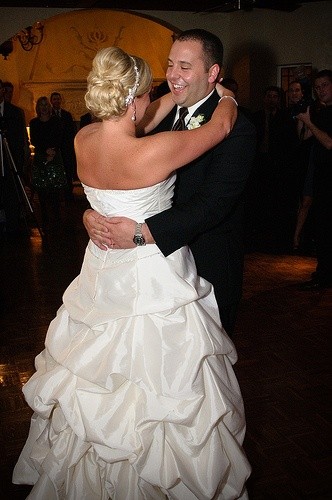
[186,114,205,130]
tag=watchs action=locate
[134,223,146,247]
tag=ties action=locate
[268,112,274,158]
[57,110,60,118]
[0,106,2,118]
[172,107,189,131]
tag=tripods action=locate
[0,117,49,248]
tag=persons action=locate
[0,79,31,224]
[31,92,76,202]
[250,81,312,240]
[292,69,332,291]
[221,77,241,106]
[31,146,66,228]
[10,47,252,500]
[82,29,239,383]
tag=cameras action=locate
[295,100,307,113]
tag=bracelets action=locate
[217,96,239,108]
